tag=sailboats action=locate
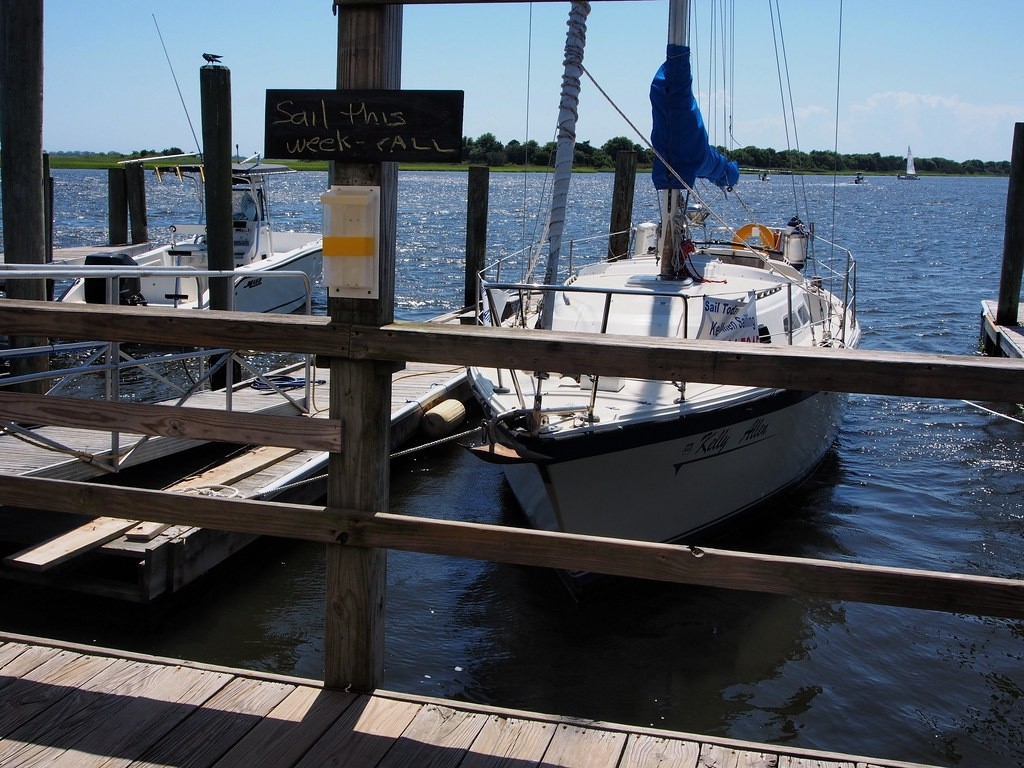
[897,144,920,180]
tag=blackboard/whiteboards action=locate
[264,87,465,165]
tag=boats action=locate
[855,172,868,184]
[755,173,772,184]
[54,144,324,314]
[464,0,862,578]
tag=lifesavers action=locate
[731,222,775,255]
